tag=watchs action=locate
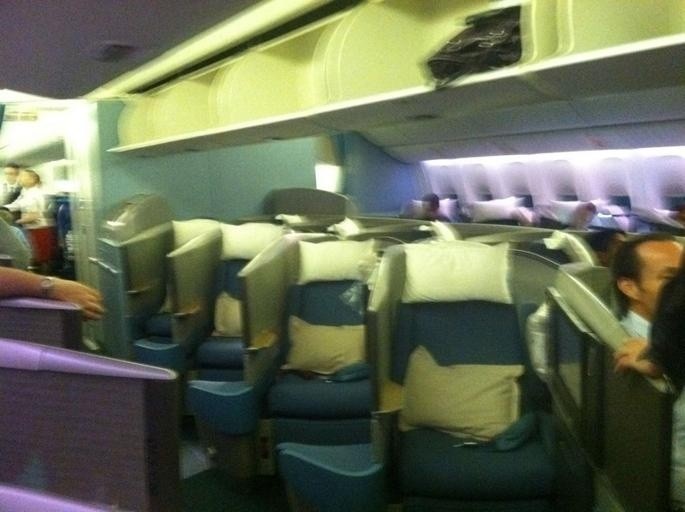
[39,276,56,298]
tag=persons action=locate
[0,263,105,320]
[610,229,685,512]
[421,192,685,236]
[0,163,61,270]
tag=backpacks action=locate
[428,6,521,89]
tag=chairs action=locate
[118,189,685,512]
